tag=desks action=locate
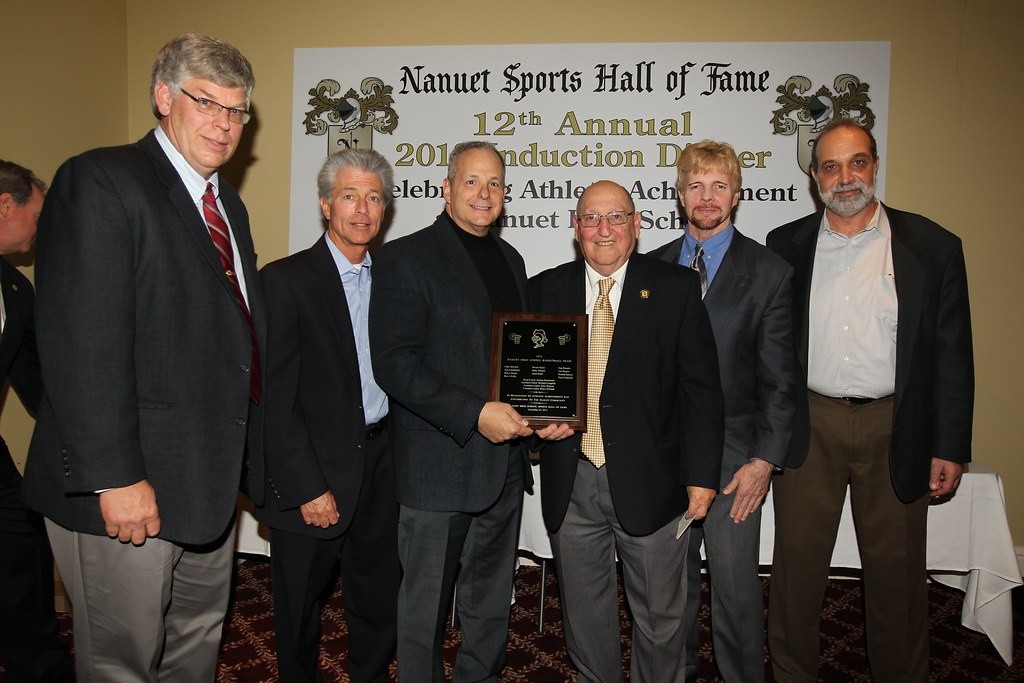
[233,457,1022,667]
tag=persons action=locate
[644,140,803,683]
[0,32,253,683]
[526,181,726,683]
[765,119,975,683]
[256,147,401,683]
[367,142,534,683]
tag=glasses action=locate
[577,210,636,228]
[179,86,255,125]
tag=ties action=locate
[688,244,707,300]
[201,181,263,407]
[581,276,617,470]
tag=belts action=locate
[365,413,392,441]
[579,451,589,461]
[841,393,896,405]
